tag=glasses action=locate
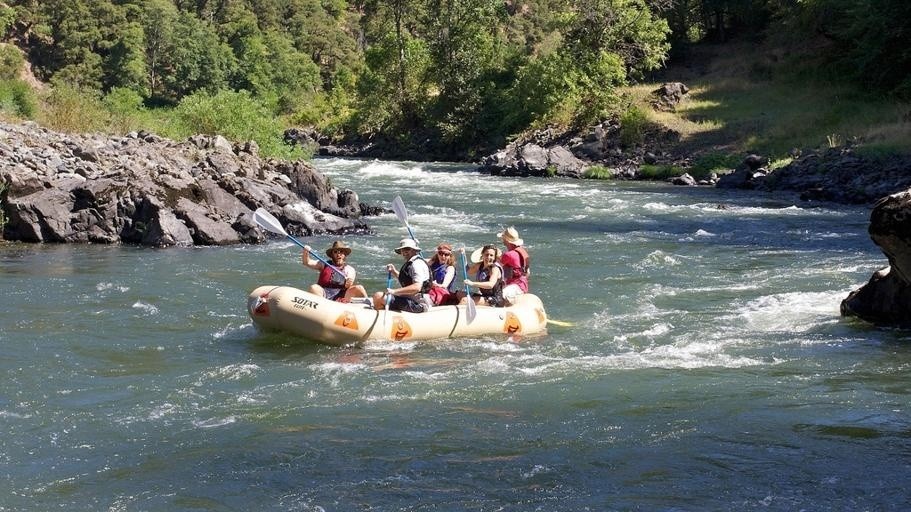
[439,251,450,256]
[334,250,344,254]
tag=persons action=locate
[372,238,434,310]
[302,240,369,301]
[496,227,531,306]
[458,243,505,306]
[422,242,458,307]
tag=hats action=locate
[326,241,351,258]
[497,227,523,246]
[395,239,421,254]
[470,247,502,264]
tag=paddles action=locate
[545,319,579,327]
[392,196,424,259]
[462,249,476,324]
[384,270,392,339]
[253,207,348,280]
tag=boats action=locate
[249,283,546,344]
